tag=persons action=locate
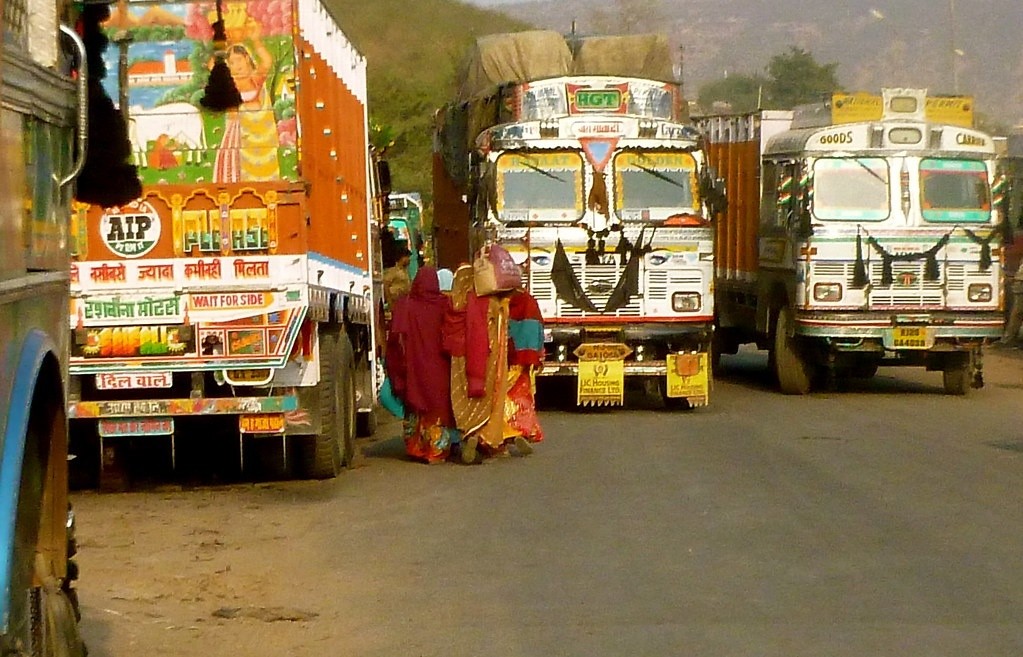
[385,244,451,464]
[993,262,1023,348]
[385,248,413,309]
[442,263,547,464]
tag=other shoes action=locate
[504,438,520,457]
[461,436,480,464]
[514,437,534,456]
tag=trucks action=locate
[432,29,728,407]
[0,0,142,657]
[64,1,393,489]
[689,88,1005,395]
[382,192,425,283]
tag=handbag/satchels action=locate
[378,377,405,420]
[473,244,515,297]
[488,244,521,288]
